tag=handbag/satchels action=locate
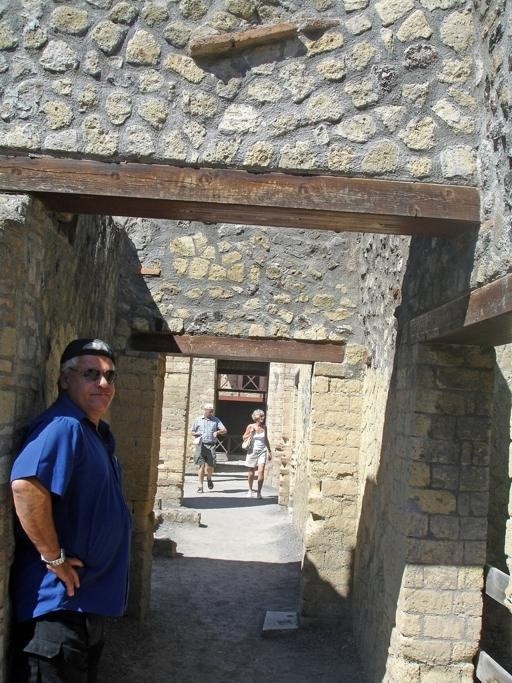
[242,423,255,450]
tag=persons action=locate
[240,407,273,499]
[190,401,227,492]
[9,333,133,682]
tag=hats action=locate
[60,338,115,363]
[204,403,214,409]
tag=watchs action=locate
[41,547,66,567]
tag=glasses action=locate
[67,365,115,384]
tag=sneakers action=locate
[208,479,213,488]
[257,493,262,498]
[197,486,203,492]
[248,491,252,497]
